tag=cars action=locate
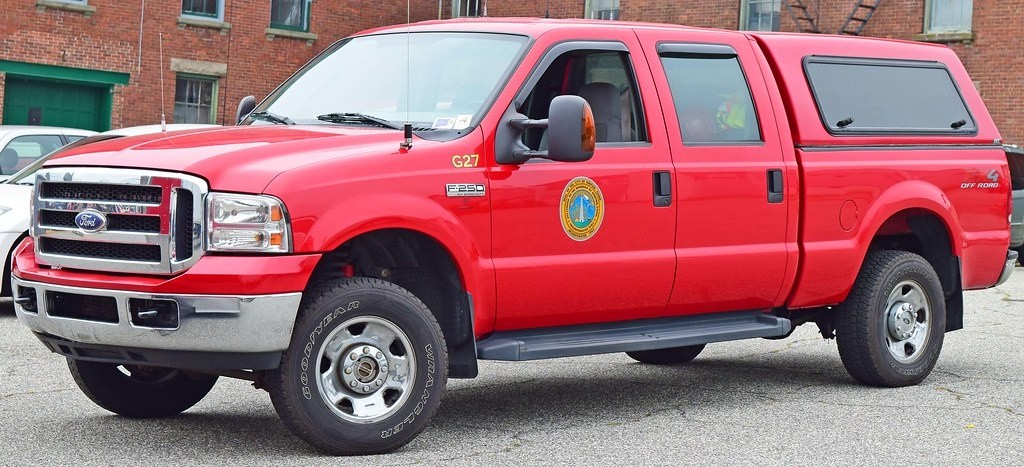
[0,125,99,295]
[1003,143,1024,268]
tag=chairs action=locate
[539,82,631,150]
[0,149,19,175]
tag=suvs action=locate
[11,17,1019,456]
